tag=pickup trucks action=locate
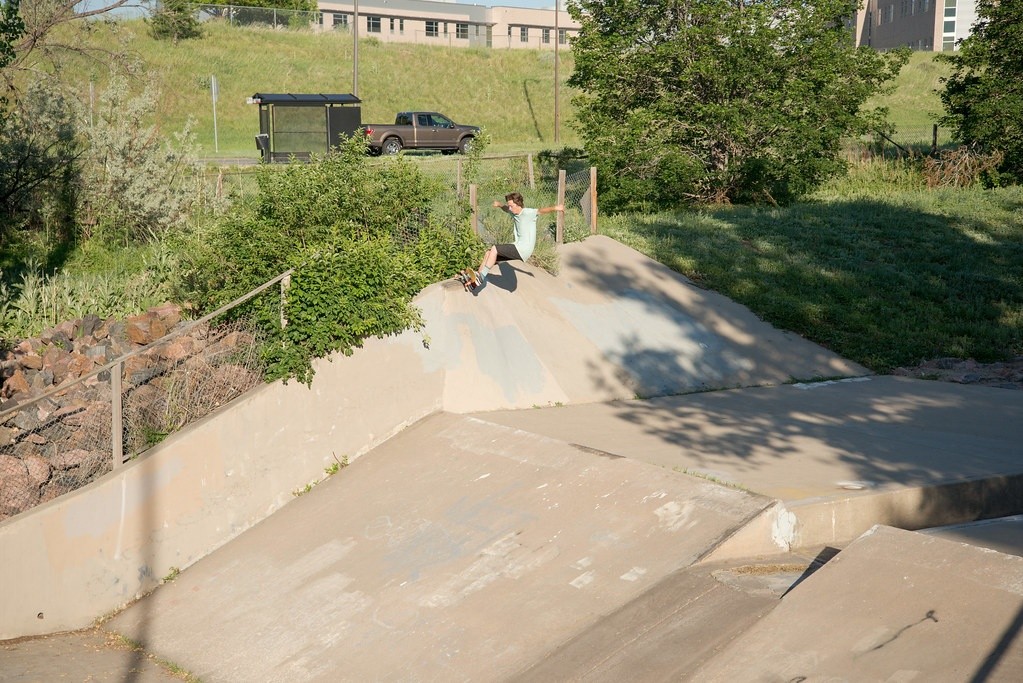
[361,111,483,157]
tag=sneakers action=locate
[462,271,475,291]
[473,270,482,286]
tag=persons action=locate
[463,193,568,291]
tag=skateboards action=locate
[454,268,476,286]
